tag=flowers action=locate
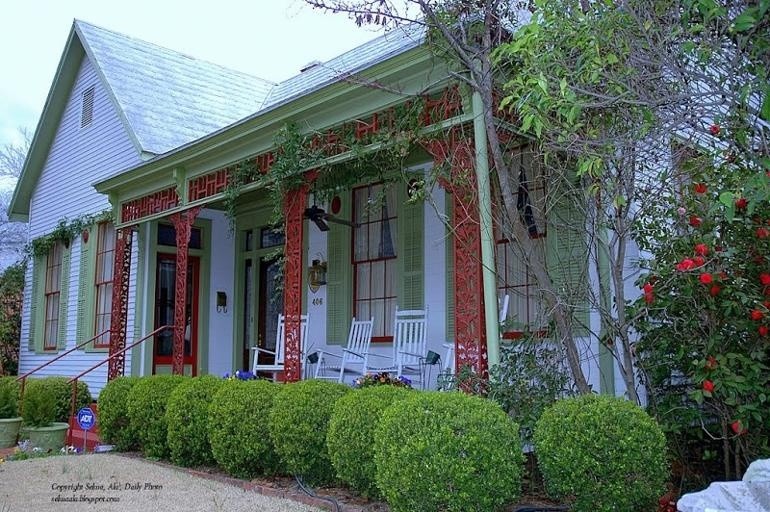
[221,368,256,381]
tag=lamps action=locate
[307,259,327,293]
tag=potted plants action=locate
[0,372,71,456]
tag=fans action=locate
[303,181,360,231]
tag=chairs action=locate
[250,294,511,392]
